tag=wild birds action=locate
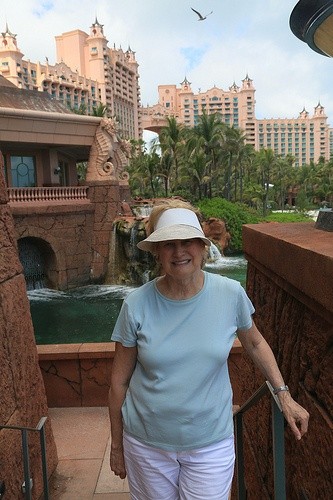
[190,8,214,21]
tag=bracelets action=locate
[273,385,289,394]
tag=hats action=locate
[136,208,211,252]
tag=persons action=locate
[108,198,309,500]
